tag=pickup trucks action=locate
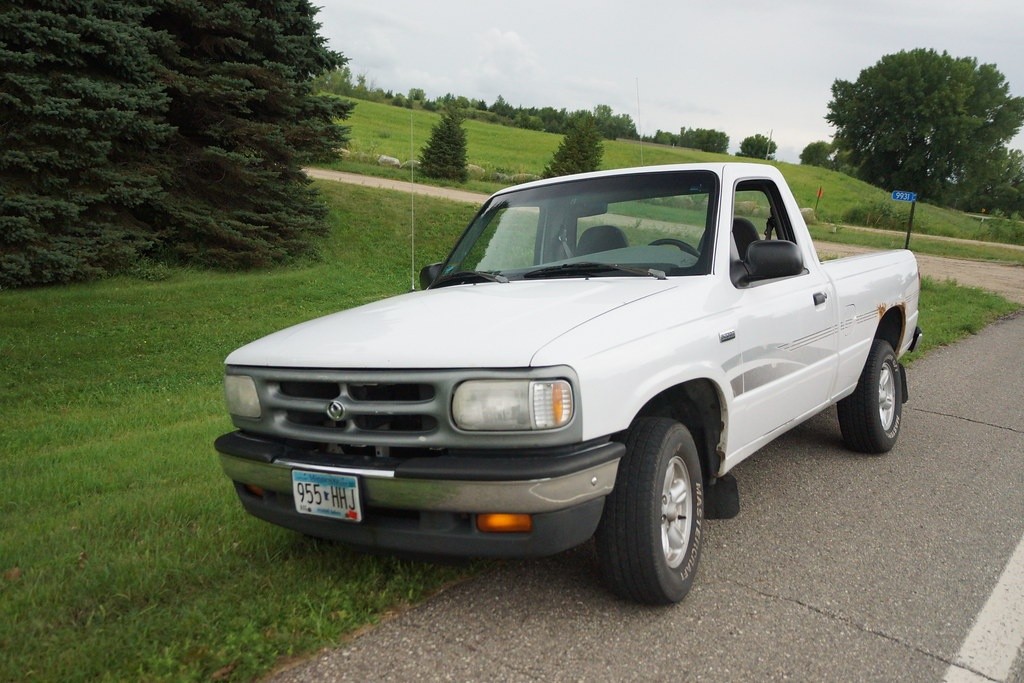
[212,161,924,607]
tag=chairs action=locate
[573,225,630,274]
[701,218,762,274]
